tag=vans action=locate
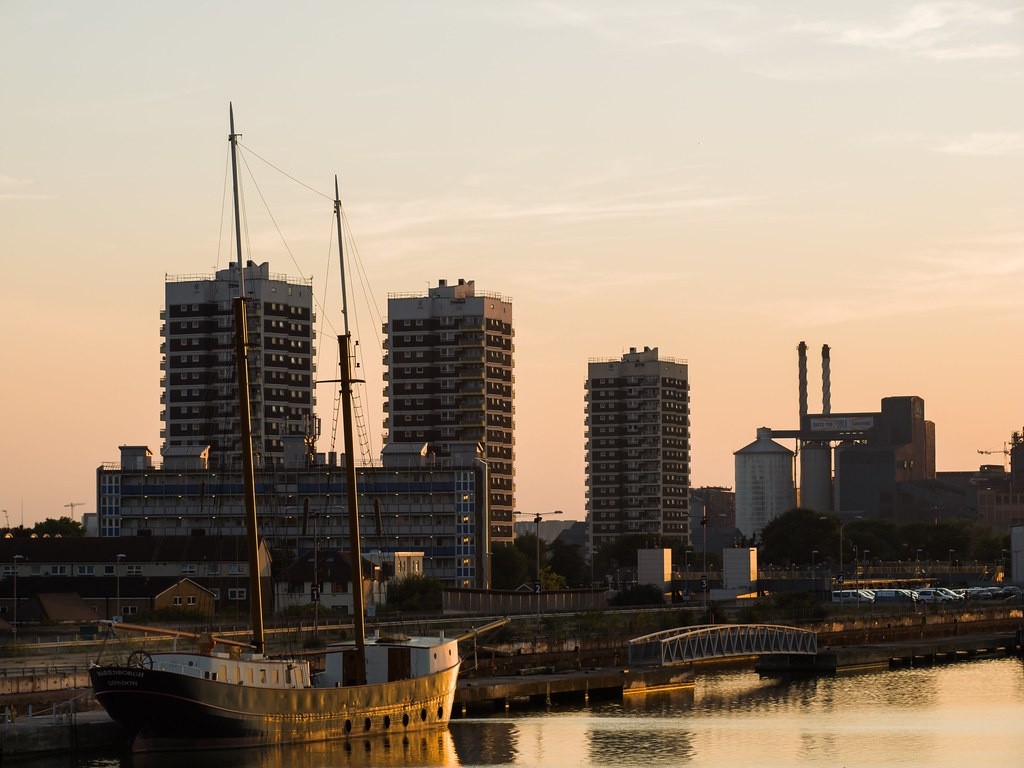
[875,590,917,605]
[920,588,965,602]
[832,590,874,605]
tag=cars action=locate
[918,590,952,605]
[951,586,1022,599]
[860,589,919,598]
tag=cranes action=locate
[977,442,1010,472]
[65,503,86,520]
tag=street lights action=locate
[863,550,869,587]
[1001,549,1007,578]
[13,554,24,643]
[916,549,922,584]
[949,549,955,588]
[685,551,692,589]
[769,564,772,580]
[681,514,728,613]
[284,506,345,637]
[117,553,126,621]
[709,564,713,580]
[812,551,819,603]
[821,515,862,606]
[1015,550,1021,584]
[747,549,755,611]
[589,550,598,609]
[853,545,859,608]
[686,564,690,577]
[606,576,610,591]
[369,552,379,621]
[792,563,795,579]
[485,552,493,614]
[515,511,564,628]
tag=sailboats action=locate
[84,99,514,752]
